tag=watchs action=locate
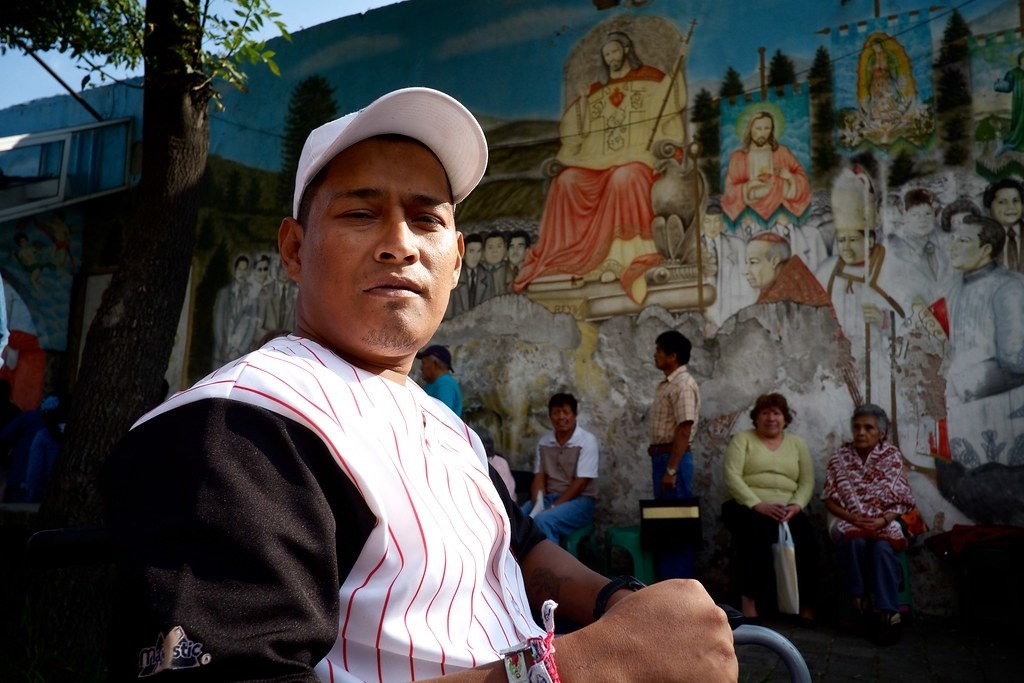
[590,575,648,622]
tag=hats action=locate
[292,87,490,227]
[417,345,454,373]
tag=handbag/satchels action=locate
[773,521,799,614]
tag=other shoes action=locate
[742,608,762,627]
[882,612,904,633]
[782,608,820,628]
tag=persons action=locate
[820,405,917,629]
[721,392,817,630]
[103,85,739,683]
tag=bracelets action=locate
[551,504,555,508]
[882,516,889,527]
[500,598,561,683]
[666,467,678,476]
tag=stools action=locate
[603,525,655,586]
[560,523,600,571]
[895,551,912,621]
